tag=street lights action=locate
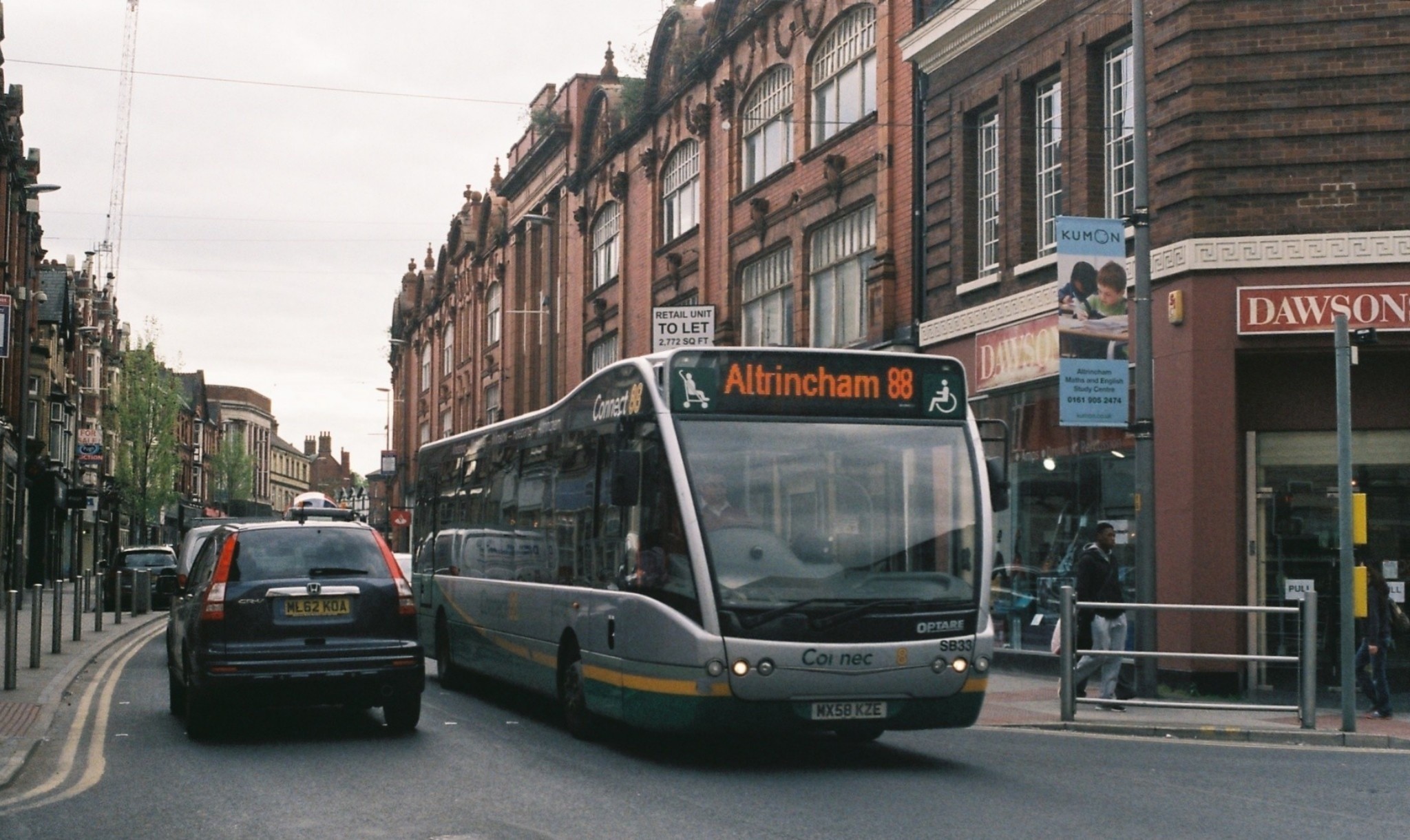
[376,386,390,545]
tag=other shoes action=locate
[1367,710,1393,720]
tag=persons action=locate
[1354,558,1396,720]
[694,467,748,533]
[1060,259,1125,358]
[1072,523,1133,712]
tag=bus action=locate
[408,343,1013,757]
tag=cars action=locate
[991,563,1060,642]
[155,511,425,739]
[160,515,283,588]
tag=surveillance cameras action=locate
[38,292,48,304]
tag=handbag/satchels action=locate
[1387,594,1410,642]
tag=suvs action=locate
[98,544,178,610]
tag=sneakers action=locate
[1095,702,1123,712]
[1057,678,1062,701]
